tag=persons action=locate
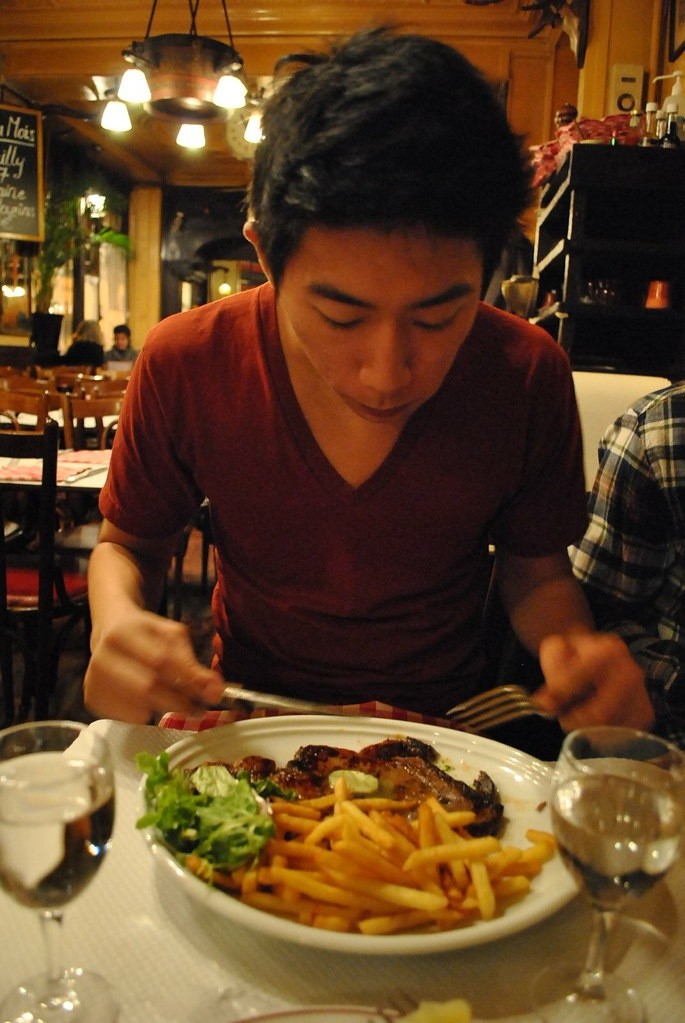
[84,18,685,768]
[104,325,139,361]
[62,320,105,366]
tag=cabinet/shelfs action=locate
[526,144,685,383]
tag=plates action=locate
[140,715,582,954]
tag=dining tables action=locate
[0,720,685,1023]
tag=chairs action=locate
[0,364,129,450]
[0,417,91,729]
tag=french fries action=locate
[183,778,560,934]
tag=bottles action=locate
[611,102,680,148]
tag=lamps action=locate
[101,0,265,149]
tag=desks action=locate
[0,407,120,494]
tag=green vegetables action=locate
[132,748,302,890]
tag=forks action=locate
[446,684,555,726]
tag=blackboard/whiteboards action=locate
[0,104,44,242]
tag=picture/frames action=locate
[669,0,685,62]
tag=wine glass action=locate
[532,725,685,1023]
[0,719,118,1022]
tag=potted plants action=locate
[29,185,135,356]
